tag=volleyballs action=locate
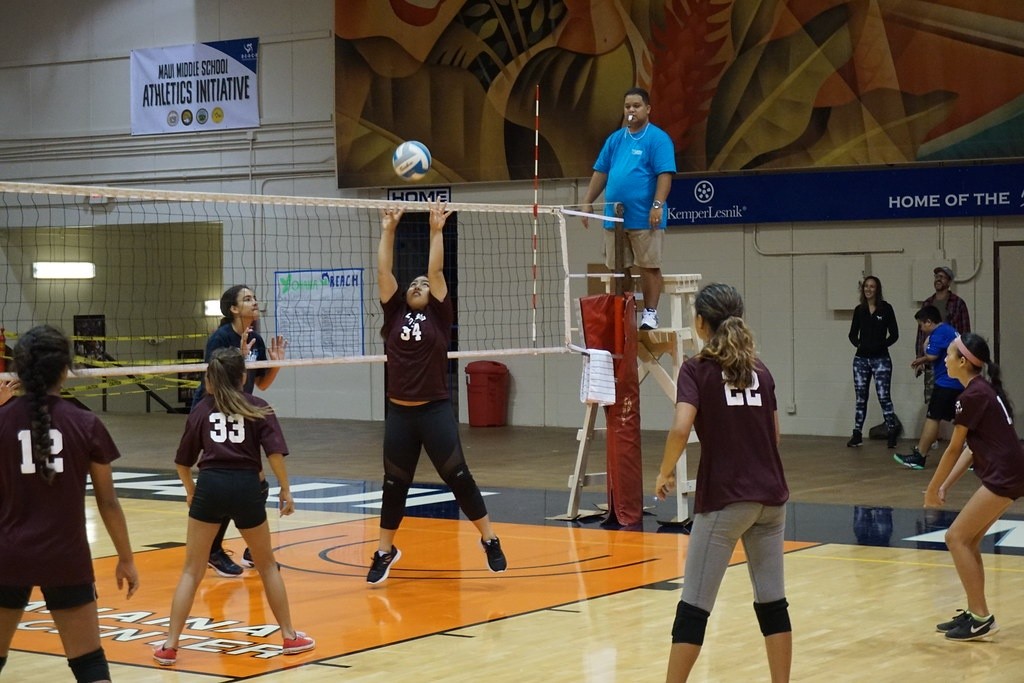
[392,141,432,183]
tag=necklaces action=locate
[625,117,650,140]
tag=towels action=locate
[579,348,616,408]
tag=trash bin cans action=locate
[465,360,510,427]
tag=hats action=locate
[934,267,954,279]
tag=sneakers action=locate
[887,429,896,449]
[208,548,244,577]
[239,548,280,570]
[639,308,658,330]
[153,643,177,666]
[893,447,925,471]
[481,535,507,574]
[846,429,862,448]
[936,610,972,633]
[366,545,401,583]
[945,615,999,641]
[283,636,316,655]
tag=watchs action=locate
[653,200,663,209]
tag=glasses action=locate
[934,275,949,281]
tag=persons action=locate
[190,285,288,578]
[580,87,676,330]
[914,266,971,450]
[366,195,508,585]
[847,276,899,449]
[654,283,792,683]
[153,346,316,666]
[0,327,13,373]
[0,325,140,683]
[893,305,980,470]
[930,333,1024,641]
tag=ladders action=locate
[565,274,701,522]
